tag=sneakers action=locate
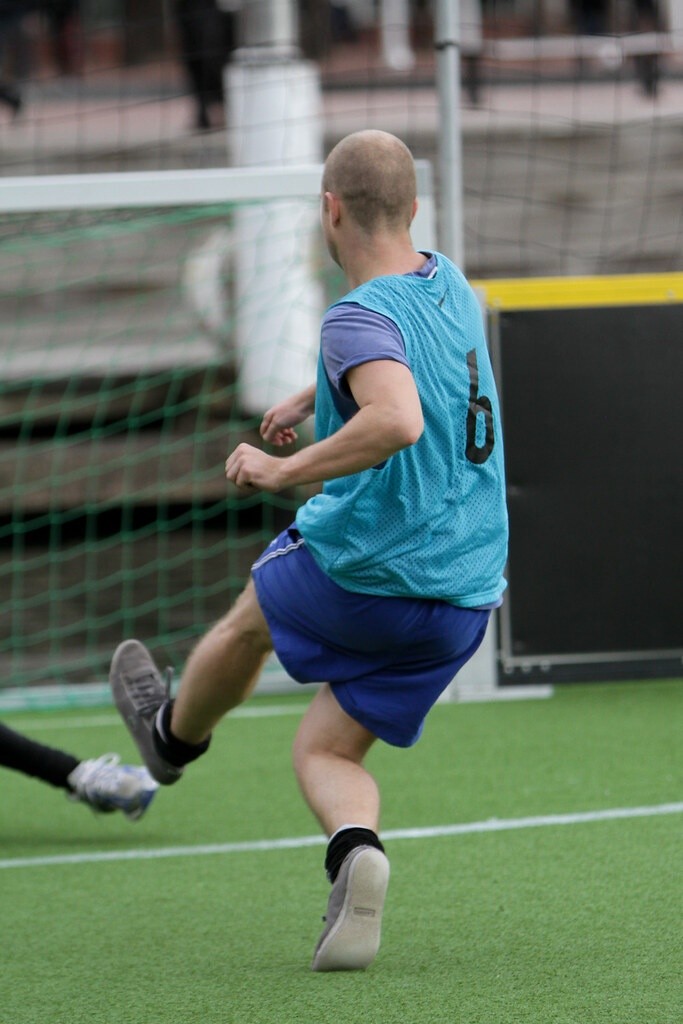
[310,843,390,971]
[109,640,186,786]
[75,755,160,821]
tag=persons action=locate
[108,129,508,974]
[0,0,668,132]
[0,724,161,823]
